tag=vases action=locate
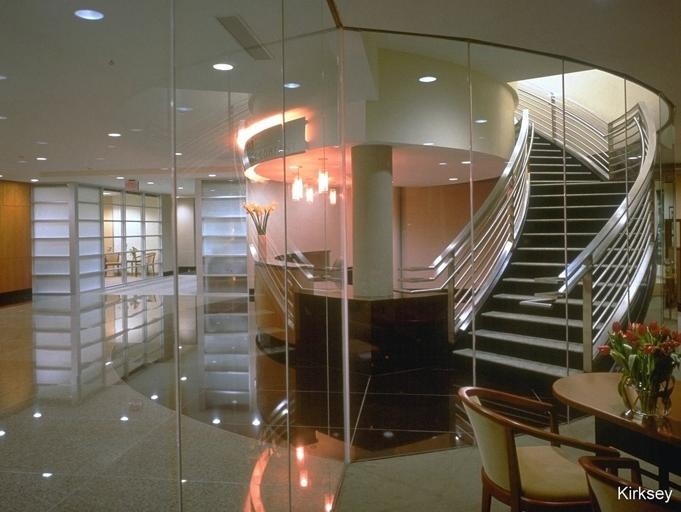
[624,373,676,423]
[258,234,268,260]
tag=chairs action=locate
[458,383,680,511]
[103,252,156,277]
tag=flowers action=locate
[606,320,680,412]
[242,200,278,236]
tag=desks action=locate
[551,370,680,498]
